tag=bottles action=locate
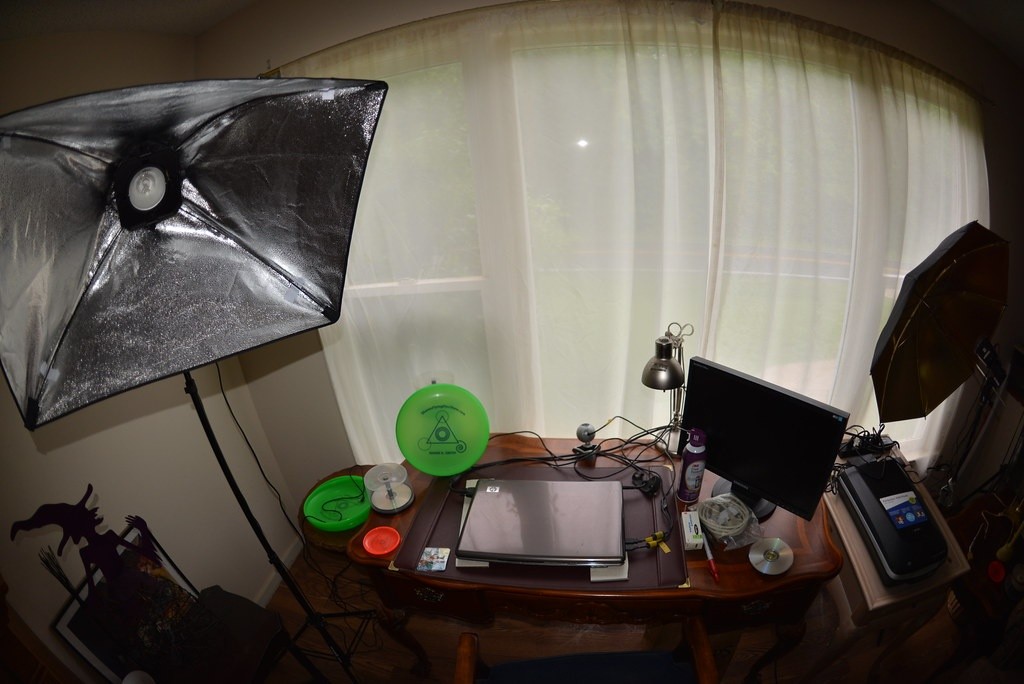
[676,427,706,503]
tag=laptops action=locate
[455,478,626,568]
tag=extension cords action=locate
[838,435,894,459]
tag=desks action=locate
[298,465,377,556]
[347,431,843,672]
[147,585,331,684]
[799,436,970,684]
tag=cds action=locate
[749,538,794,575]
[371,481,411,509]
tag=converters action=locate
[847,454,877,467]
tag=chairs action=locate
[452,615,719,684]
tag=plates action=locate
[363,526,402,558]
[988,561,1004,583]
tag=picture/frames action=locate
[49,521,200,684]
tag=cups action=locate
[121,670,155,684]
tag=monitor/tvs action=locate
[676,355,850,524]
